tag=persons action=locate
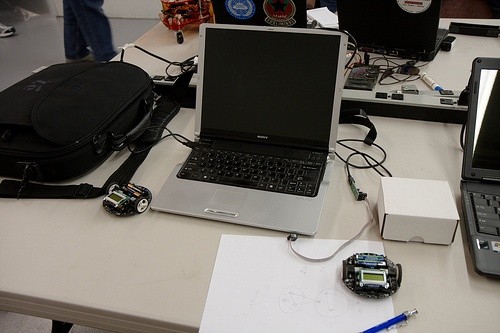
[63,0,119,62]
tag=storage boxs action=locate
[377,175,460,246]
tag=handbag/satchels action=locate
[0,62,155,201]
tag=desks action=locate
[0,18,500,333]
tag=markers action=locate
[420,72,442,91]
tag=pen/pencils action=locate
[361,309,418,333]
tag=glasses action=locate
[379,63,420,85]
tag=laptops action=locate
[336,0,450,61]
[459,58,500,277]
[150,23,348,236]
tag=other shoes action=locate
[66,54,96,62]
[0,23,15,37]
